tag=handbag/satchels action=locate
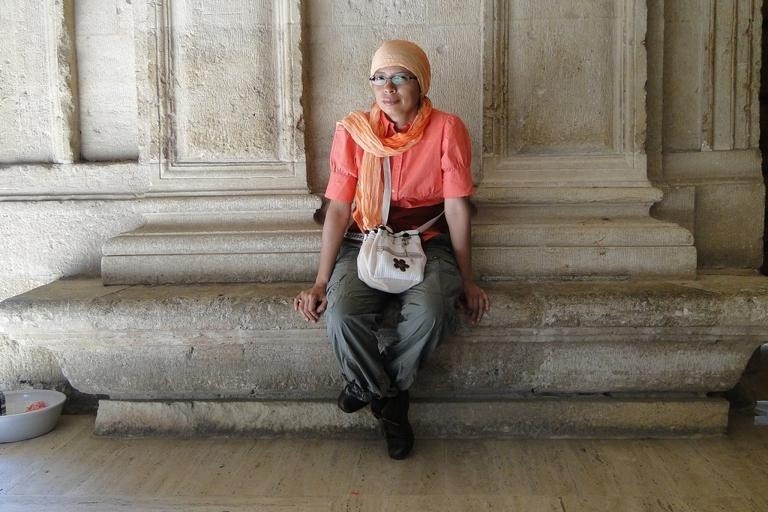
[355,223,427,293]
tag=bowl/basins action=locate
[0,390,67,443]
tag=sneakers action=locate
[338,379,370,413]
[381,387,415,460]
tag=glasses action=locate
[369,74,417,86]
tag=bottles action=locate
[0,391,6,416]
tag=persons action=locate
[292,39,491,461]
